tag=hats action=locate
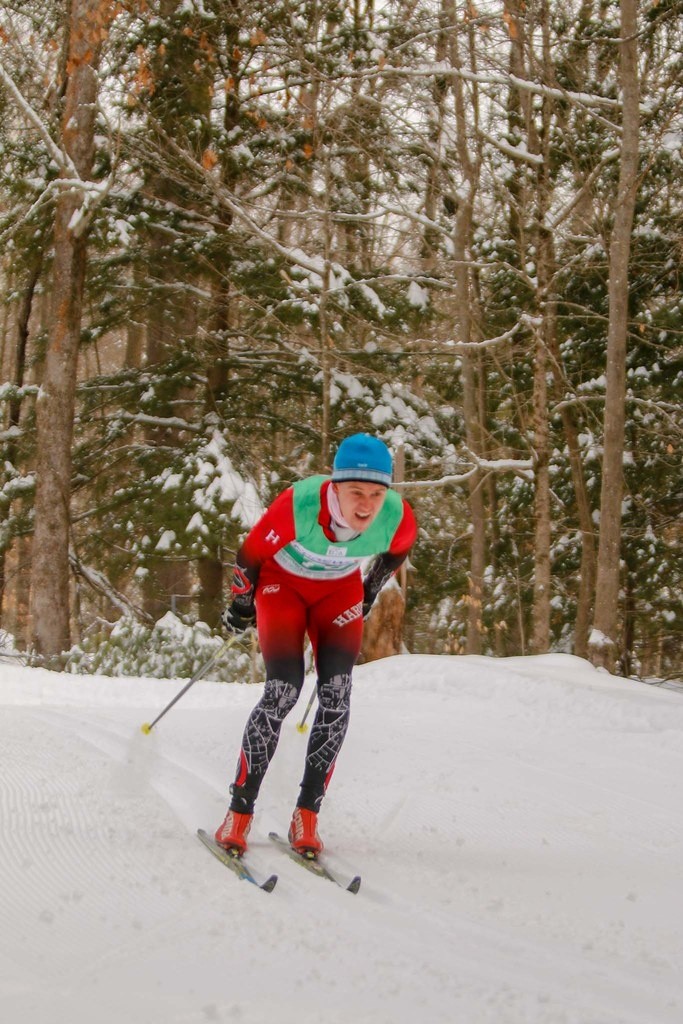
[332,432,392,490]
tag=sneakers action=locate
[288,807,324,859]
[214,808,254,857]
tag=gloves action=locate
[222,601,257,634]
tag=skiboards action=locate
[196,827,362,894]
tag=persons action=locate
[215,433,417,860]
